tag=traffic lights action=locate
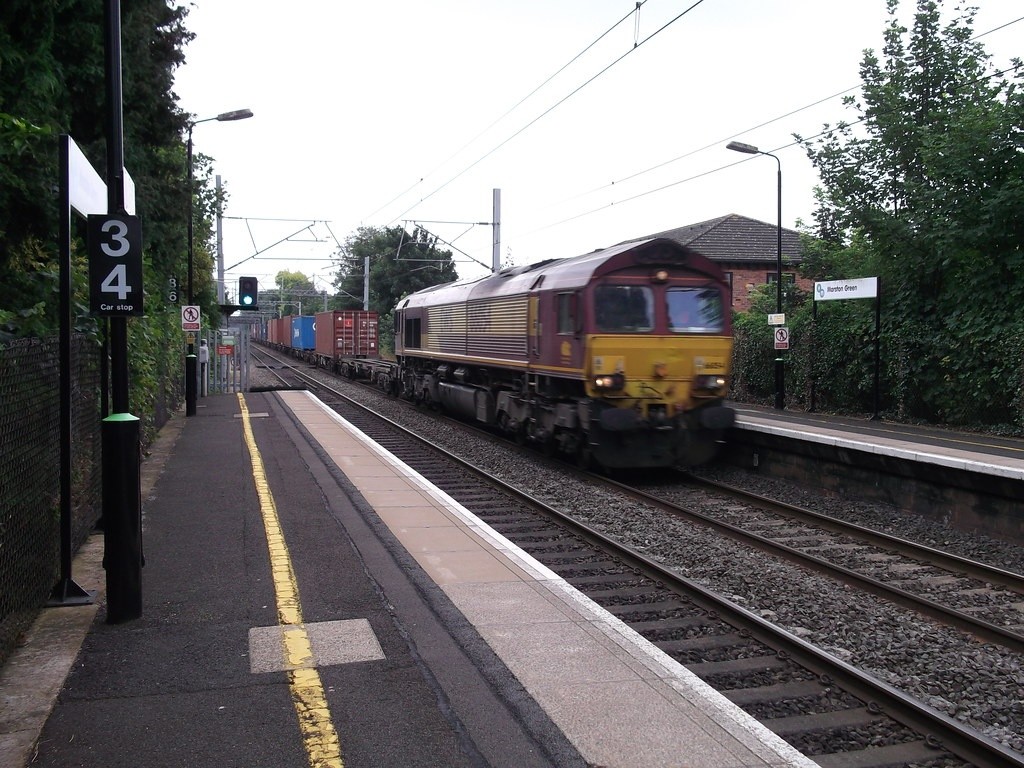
[239,276,258,307]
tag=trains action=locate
[250,236,739,478]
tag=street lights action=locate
[184,107,255,417]
[724,141,786,410]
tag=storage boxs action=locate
[247,309,379,360]
